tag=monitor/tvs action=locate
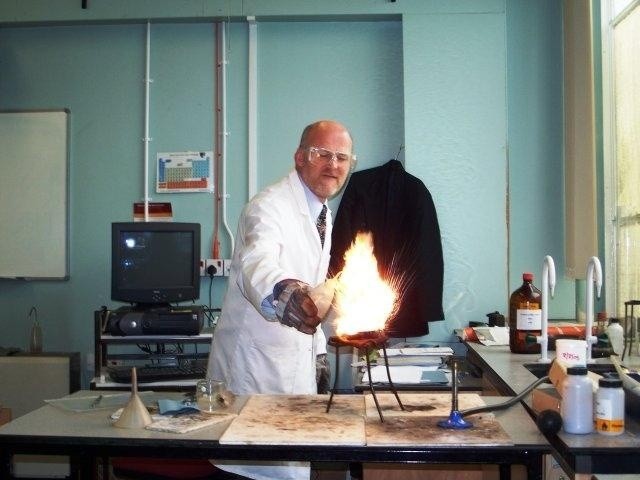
[112,222,200,312]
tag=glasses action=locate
[300,143,357,173]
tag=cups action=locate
[554,339,587,368]
[197,380,226,410]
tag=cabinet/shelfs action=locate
[94,306,222,393]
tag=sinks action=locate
[521,362,639,384]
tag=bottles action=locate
[509,273,545,355]
[563,367,625,435]
[305,279,335,321]
[31,321,43,355]
[596,313,625,356]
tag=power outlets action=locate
[205,257,223,278]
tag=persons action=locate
[205,120,356,480]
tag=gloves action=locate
[273,279,321,334]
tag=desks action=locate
[1,388,555,480]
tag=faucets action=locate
[525,254,555,364]
[584,255,603,362]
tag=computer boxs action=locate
[110,307,204,335]
